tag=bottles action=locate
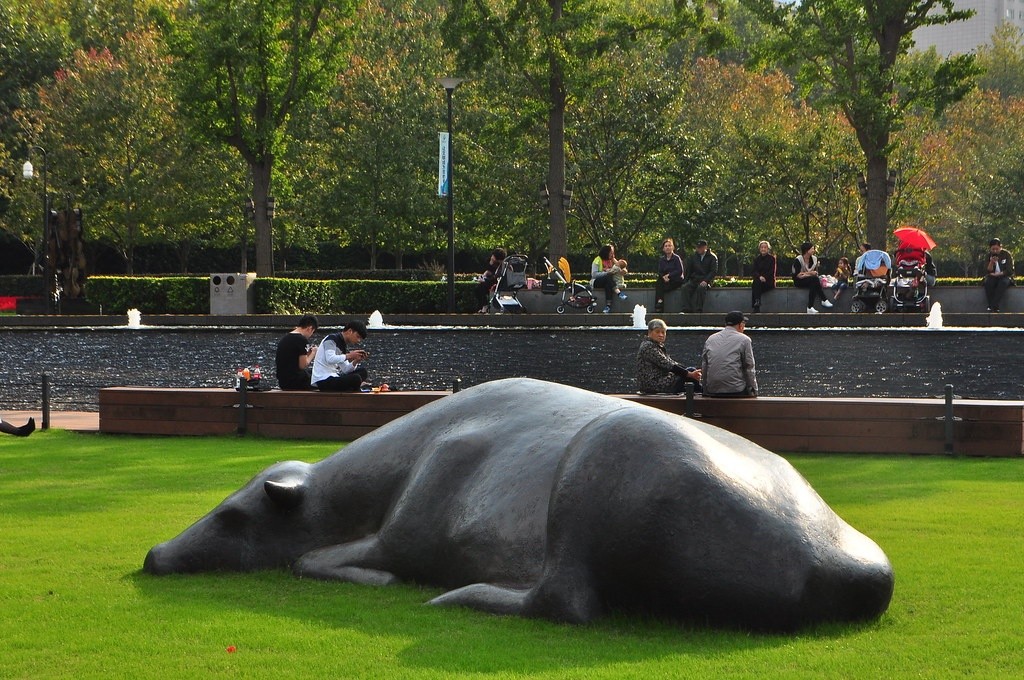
[253,365,260,380]
[243,368,250,381]
[236,369,242,388]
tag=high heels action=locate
[477,305,490,314]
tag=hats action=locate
[726,311,749,326]
[693,240,707,246]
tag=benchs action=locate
[96,384,1024,457]
[486,286,1024,314]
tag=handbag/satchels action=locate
[542,279,558,295]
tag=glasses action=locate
[741,321,746,325]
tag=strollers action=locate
[479,254,530,313]
[542,257,598,315]
[889,248,931,313]
[851,250,892,313]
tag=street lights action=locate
[438,76,465,313]
[23,146,52,315]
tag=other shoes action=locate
[834,295,838,301]
[986,307,992,312]
[995,309,1000,313]
[603,306,610,313]
[618,292,627,300]
[754,300,762,307]
[752,308,760,313]
[654,302,663,308]
[18,418,36,437]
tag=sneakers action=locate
[806,307,819,313]
[822,299,834,307]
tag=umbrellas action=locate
[893,227,936,250]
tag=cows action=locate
[144,375,896,631]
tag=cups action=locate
[684,382,694,400]
[453,378,461,393]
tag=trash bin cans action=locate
[209,273,257,314]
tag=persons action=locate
[635,319,703,395]
[311,320,370,392]
[276,313,319,390]
[654,238,684,312]
[854,243,873,276]
[751,241,777,313]
[924,252,936,285]
[832,257,851,301]
[478,248,513,313]
[983,238,1015,314]
[701,311,758,398]
[589,243,627,313]
[792,243,833,314]
[681,239,718,313]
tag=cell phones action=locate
[993,254,997,257]
[359,351,366,354]
